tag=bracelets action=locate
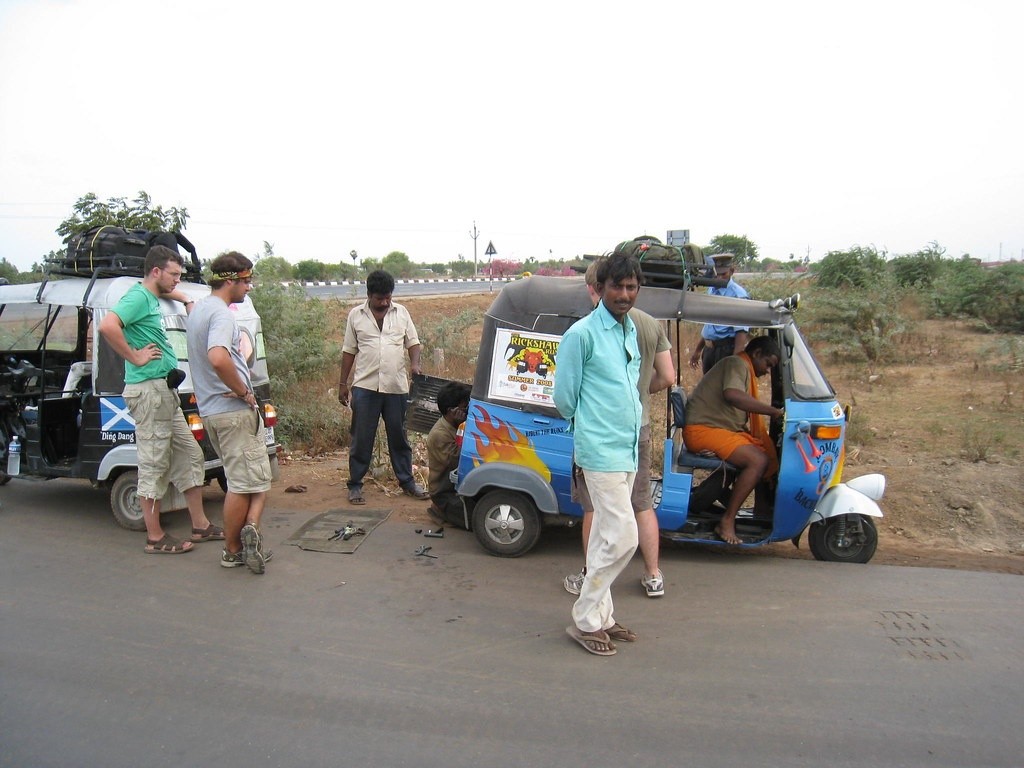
[238,391,248,397]
[184,300,194,306]
[340,383,348,385]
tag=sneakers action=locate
[222,546,273,568]
[563,565,586,594]
[641,566,664,596]
[241,524,267,574]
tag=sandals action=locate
[144,530,194,555]
[190,521,225,543]
[347,485,366,505]
[404,484,431,500]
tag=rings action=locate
[339,400,342,401]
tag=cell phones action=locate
[451,407,461,414]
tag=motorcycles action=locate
[402,236,887,564]
[0,254,284,535]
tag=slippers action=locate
[426,508,455,529]
[566,622,618,655]
[604,622,635,642]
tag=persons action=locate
[553,252,643,656]
[689,254,748,376]
[187,251,272,573]
[682,336,783,544]
[99,246,224,554]
[427,381,474,530]
[562,260,675,596]
[338,271,429,504]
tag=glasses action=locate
[155,265,182,280]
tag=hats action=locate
[708,253,734,275]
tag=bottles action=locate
[6,436,21,475]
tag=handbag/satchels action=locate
[615,235,705,281]
[67,227,199,272]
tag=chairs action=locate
[670,384,738,471]
[21,361,92,421]
